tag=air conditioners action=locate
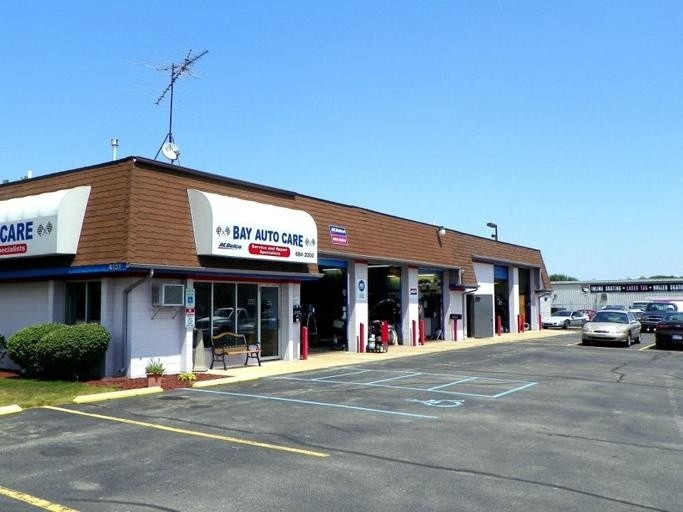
[151,283,185,307]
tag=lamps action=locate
[438,226,446,236]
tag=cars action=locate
[581,301,683,346]
[541,310,589,329]
[195,306,278,336]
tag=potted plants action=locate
[177,372,197,388]
[145,357,166,388]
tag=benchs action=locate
[210,331,263,371]
